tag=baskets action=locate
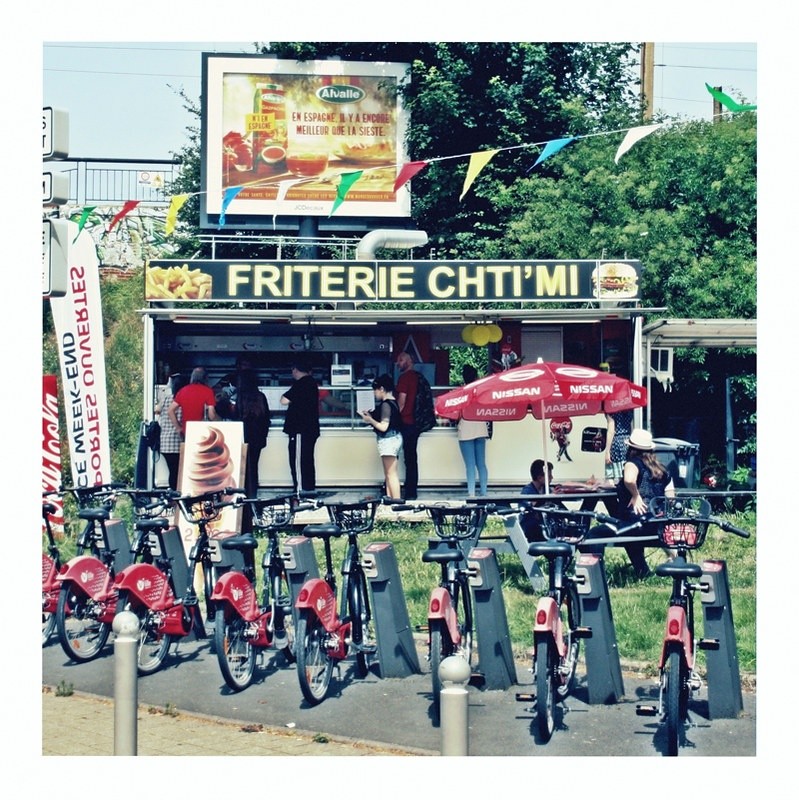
[78,488,119,511]
[138,492,180,516]
[328,500,378,533]
[542,510,587,547]
[252,497,299,531]
[186,494,224,522]
[648,495,711,547]
[436,510,477,541]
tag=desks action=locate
[450,492,673,593]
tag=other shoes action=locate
[294,490,316,497]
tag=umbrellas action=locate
[434,357,648,495]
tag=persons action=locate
[603,409,634,486]
[218,355,256,388]
[362,374,402,499]
[156,375,191,492]
[395,352,421,500]
[280,357,320,492]
[236,369,271,499]
[457,364,489,497]
[518,459,569,542]
[589,429,675,574]
[169,367,216,443]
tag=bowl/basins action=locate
[261,146,287,164]
[285,150,330,179]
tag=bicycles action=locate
[43,483,751,756]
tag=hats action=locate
[623,427,656,450]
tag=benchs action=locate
[555,530,706,545]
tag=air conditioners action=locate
[647,346,673,375]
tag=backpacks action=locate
[145,397,167,463]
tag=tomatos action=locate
[221,130,254,172]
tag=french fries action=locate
[148,262,214,301]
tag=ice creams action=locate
[189,426,237,538]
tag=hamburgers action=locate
[591,262,637,299]
